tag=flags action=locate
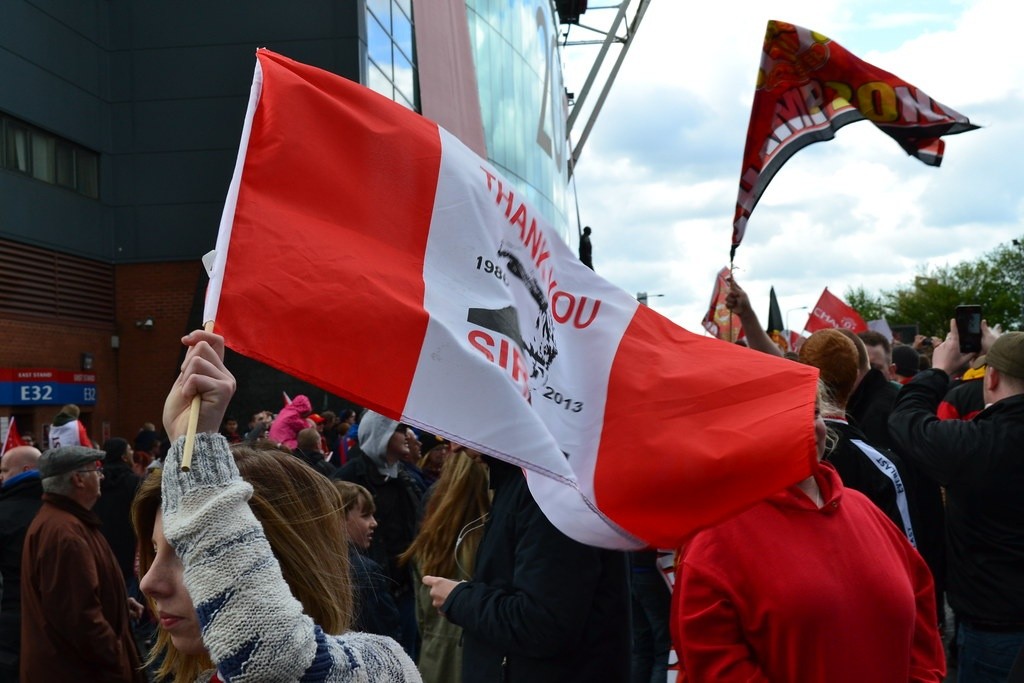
[1,418,32,457]
[767,287,800,352]
[805,289,893,344]
[702,266,746,343]
[730,19,985,261]
[203,49,820,550]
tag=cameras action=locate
[923,338,932,346]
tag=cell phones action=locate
[955,305,983,354]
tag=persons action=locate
[579,226,594,270]
[0,317,1024,683]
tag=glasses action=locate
[395,423,408,434]
[432,445,449,451]
[25,440,32,443]
[76,467,105,478]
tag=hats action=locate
[419,435,450,458]
[38,446,107,478]
[973,329,1024,377]
[308,413,326,424]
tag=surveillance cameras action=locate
[144,319,153,328]
